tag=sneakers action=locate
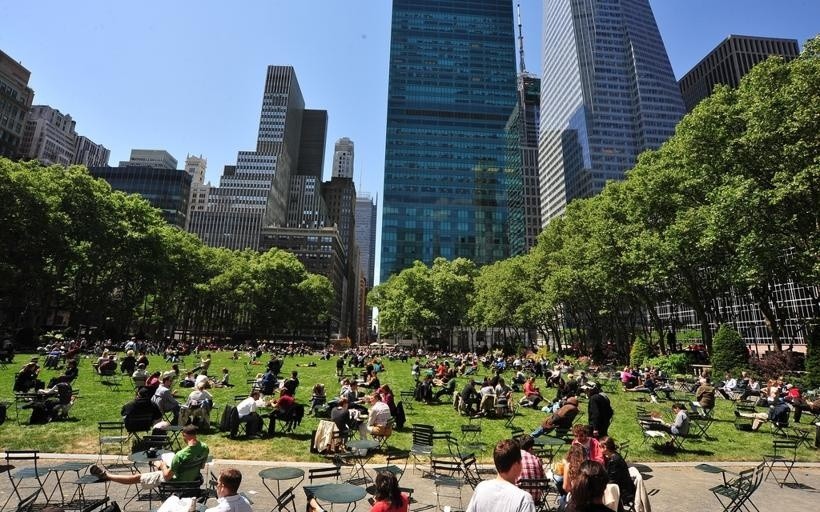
[90,465,106,480]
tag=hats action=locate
[581,380,601,392]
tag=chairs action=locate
[0,338,820,512]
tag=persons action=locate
[587,384,615,439]
[155,376,181,426]
[93,336,150,376]
[231,340,313,393]
[13,357,78,417]
[145,338,192,363]
[320,344,386,390]
[387,343,478,404]
[163,364,179,382]
[37,338,88,369]
[121,386,162,432]
[90,425,209,490]
[180,381,213,428]
[131,363,150,379]
[204,468,253,511]
[688,367,819,449]
[306,380,396,457]
[3,339,15,363]
[234,390,265,438]
[642,403,691,434]
[179,338,229,388]
[371,470,408,511]
[281,370,300,398]
[460,347,602,419]
[267,387,294,434]
[467,424,637,512]
[619,365,668,394]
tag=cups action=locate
[556,463,565,477]
[146,448,157,457]
